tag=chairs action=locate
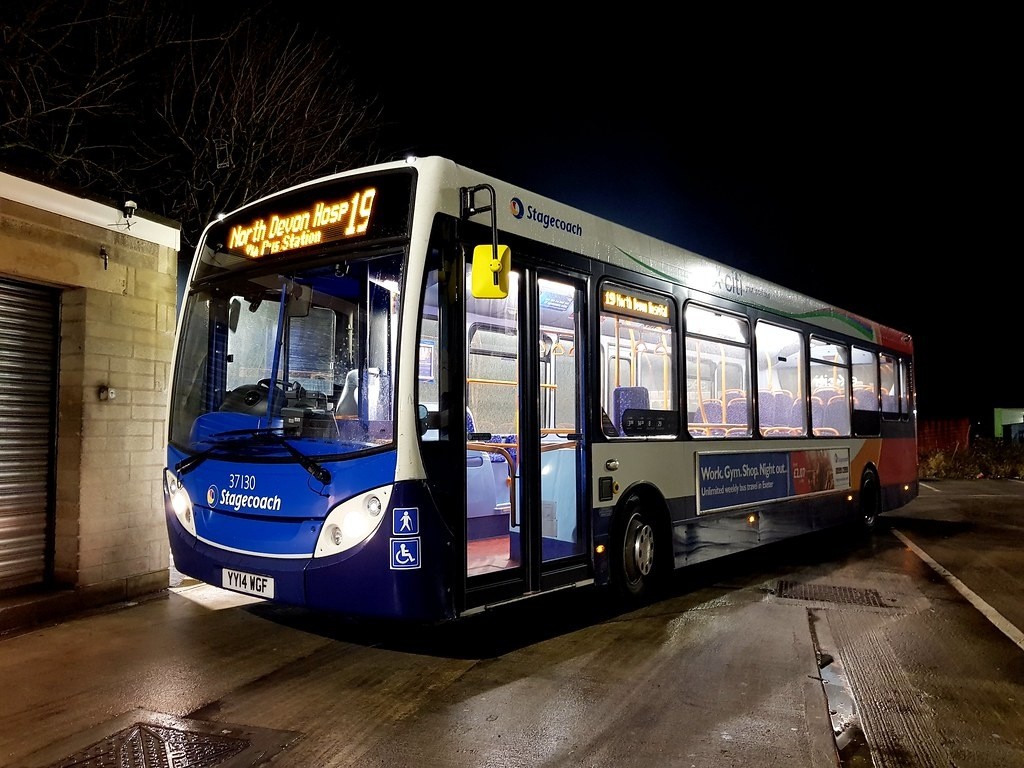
[688,385,906,438]
[337,367,387,420]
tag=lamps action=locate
[123,200,138,218]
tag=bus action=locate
[162,155,919,627]
